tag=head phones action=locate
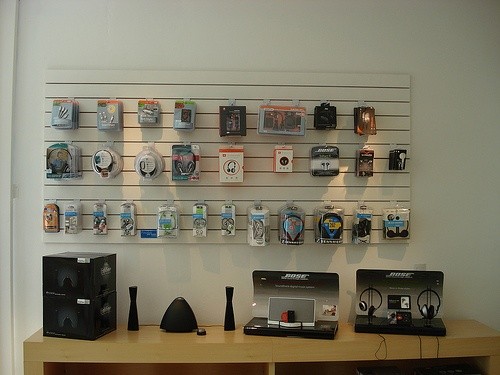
[417,289,440,319]
[227,161,236,173]
[176,160,196,176]
[48,147,73,173]
[319,212,344,239]
[359,287,383,317]
[94,150,113,173]
[283,215,304,241]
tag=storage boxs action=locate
[42,252,117,341]
[45,99,377,183]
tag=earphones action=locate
[200,230,203,234]
[196,230,198,235]
[399,153,406,163]
[321,162,325,168]
[326,163,330,169]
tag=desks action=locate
[22,319,500,375]
